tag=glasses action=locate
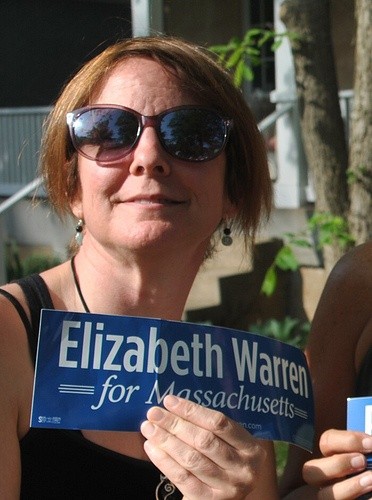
[66,104,234,163]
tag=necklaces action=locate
[69,254,92,317]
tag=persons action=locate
[0,36,282,500]
[279,241,372,500]
